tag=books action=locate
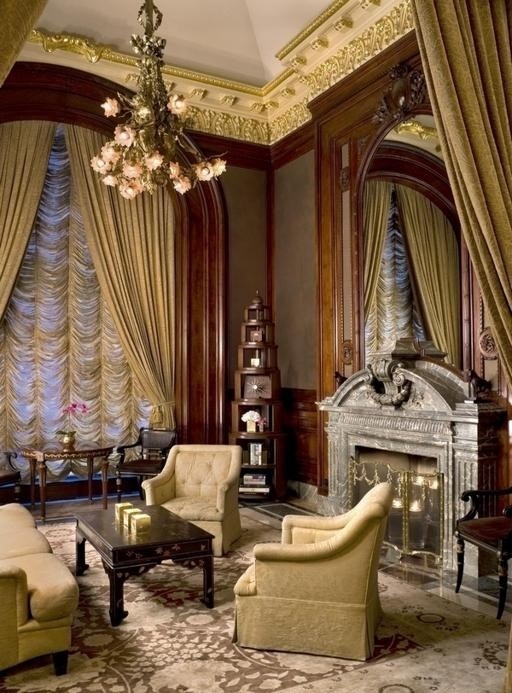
[237,440,270,492]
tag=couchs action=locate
[2,503,79,681]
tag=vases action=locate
[59,429,79,452]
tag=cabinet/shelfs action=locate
[226,292,291,500]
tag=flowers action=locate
[54,402,89,436]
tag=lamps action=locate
[92,1,229,199]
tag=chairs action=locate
[229,481,393,660]
[116,427,176,502]
[0,451,21,502]
[451,484,511,621]
[138,444,244,555]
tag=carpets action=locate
[2,493,510,693]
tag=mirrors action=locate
[354,59,475,386]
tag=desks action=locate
[24,435,114,515]
[70,502,216,626]
